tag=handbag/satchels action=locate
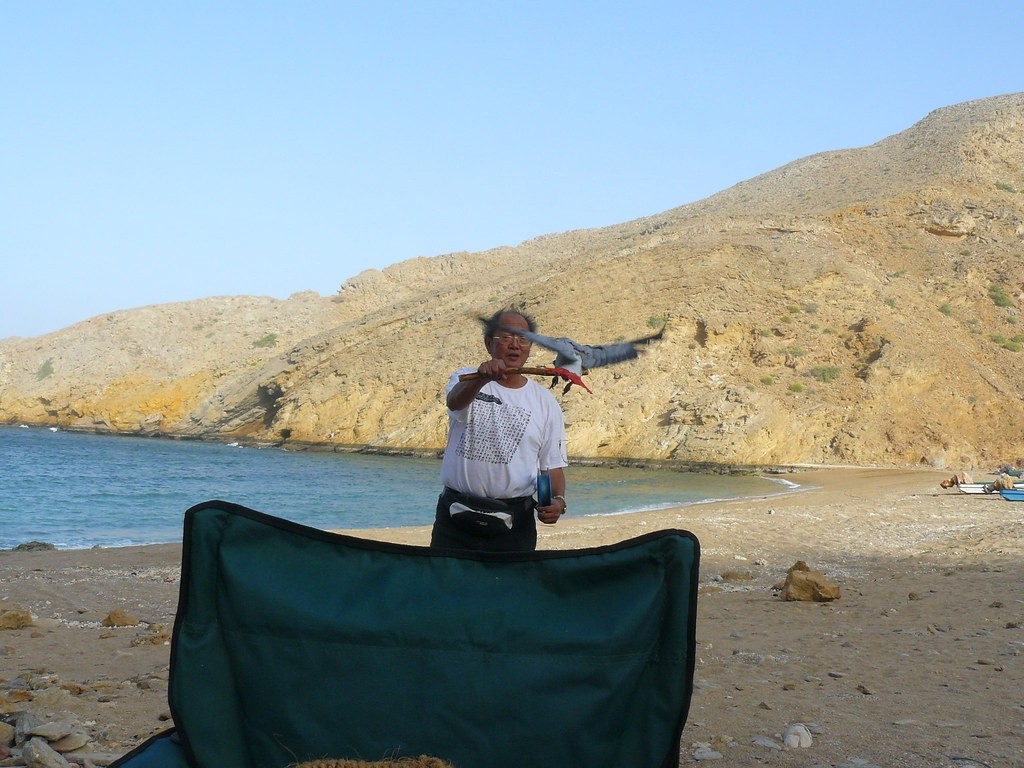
[439,487,537,536]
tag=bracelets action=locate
[553,495,567,514]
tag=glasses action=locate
[491,336,531,347]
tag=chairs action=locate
[103,501,700,768]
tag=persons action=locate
[430,308,568,552]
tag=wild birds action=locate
[476,316,662,396]
